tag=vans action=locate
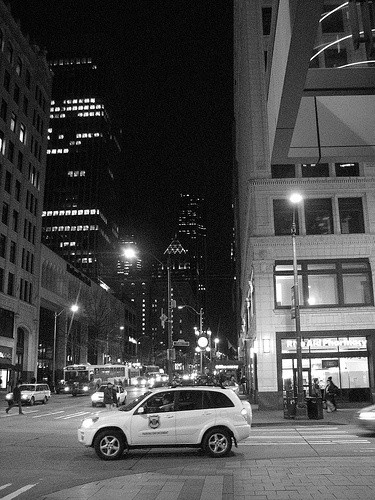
[5,384,51,405]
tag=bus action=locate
[63,362,129,397]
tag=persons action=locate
[326,381,336,413]
[5,383,24,415]
[313,379,321,398]
[104,382,114,409]
[157,394,172,412]
[112,385,118,408]
[323,377,334,409]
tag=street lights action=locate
[100,325,162,367]
[288,193,309,419]
[177,305,205,376]
[123,248,174,386]
[53,304,79,393]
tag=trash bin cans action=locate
[306,396,323,419]
[284,397,298,418]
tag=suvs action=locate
[78,385,253,458]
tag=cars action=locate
[129,363,198,390]
[350,405,375,436]
[91,384,127,406]
